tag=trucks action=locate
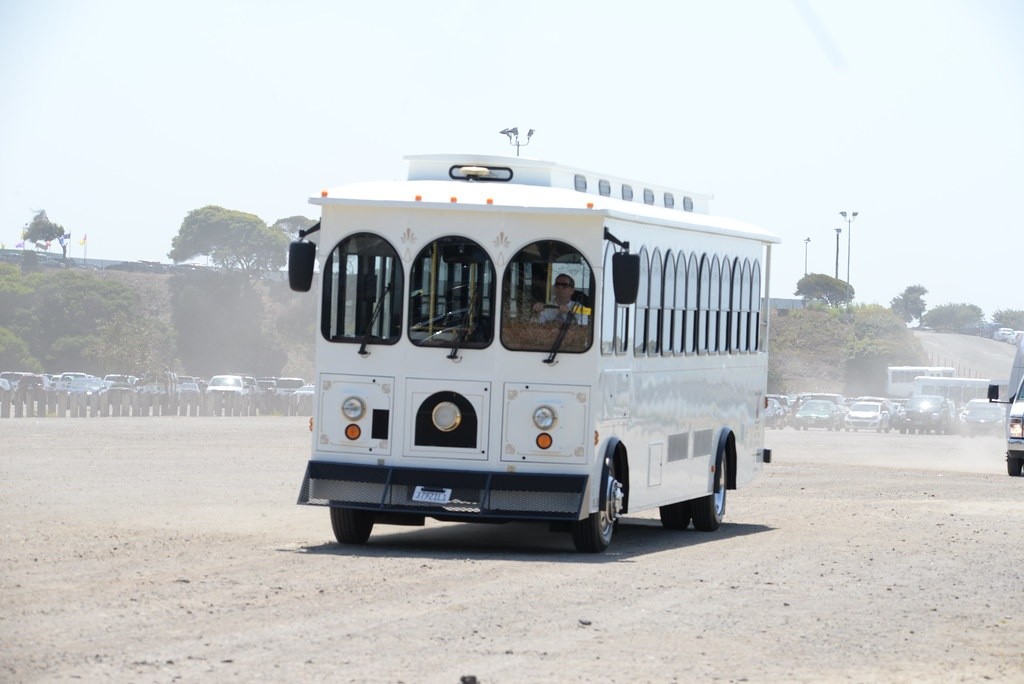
[985,337,1024,476]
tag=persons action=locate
[530,274,589,328]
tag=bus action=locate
[910,376,995,405]
[285,151,781,555]
[886,365,958,397]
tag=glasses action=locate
[555,282,572,289]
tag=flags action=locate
[16,234,86,248]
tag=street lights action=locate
[839,211,859,308]
[803,237,812,309]
[835,228,842,309]
[499,127,536,156]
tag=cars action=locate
[0,367,315,409]
[979,323,1024,346]
[764,392,1007,438]
